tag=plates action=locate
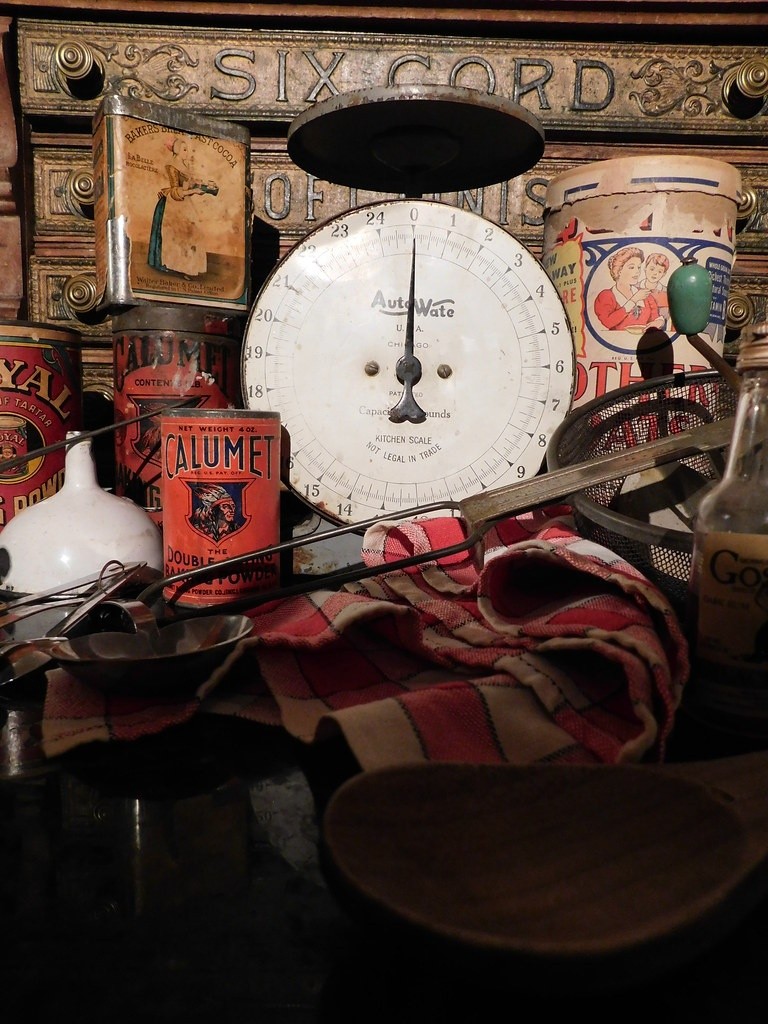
[322,755,768,957]
[47,615,255,695]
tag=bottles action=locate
[0,430,165,594]
[681,322,768,753]
